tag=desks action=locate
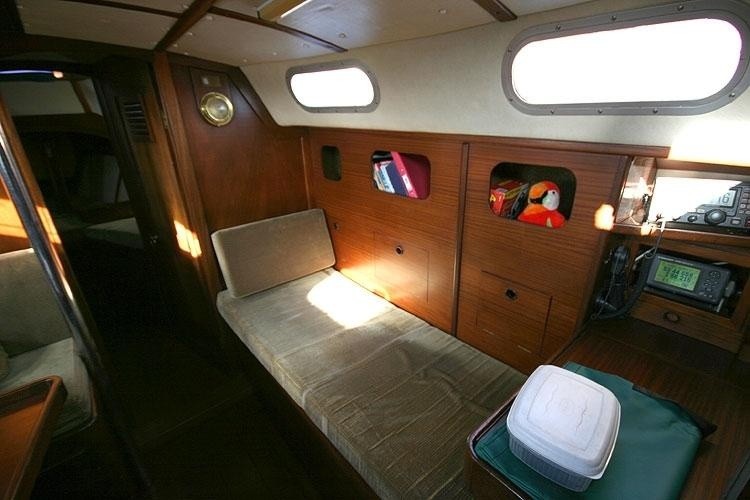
[0,376,71,500]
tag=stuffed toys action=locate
[517,178,567,229]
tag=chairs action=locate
[1,243,103,452]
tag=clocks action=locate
[199,90,235,127]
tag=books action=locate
[372,149,431,201]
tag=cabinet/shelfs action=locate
[467,317,750,500]
[293,129,357,284]
[348,134,469,335]
[457,142,660,375]
[613,223,749,362]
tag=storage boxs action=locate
[501,361,626,493]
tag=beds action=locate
[209,207,541,500]
